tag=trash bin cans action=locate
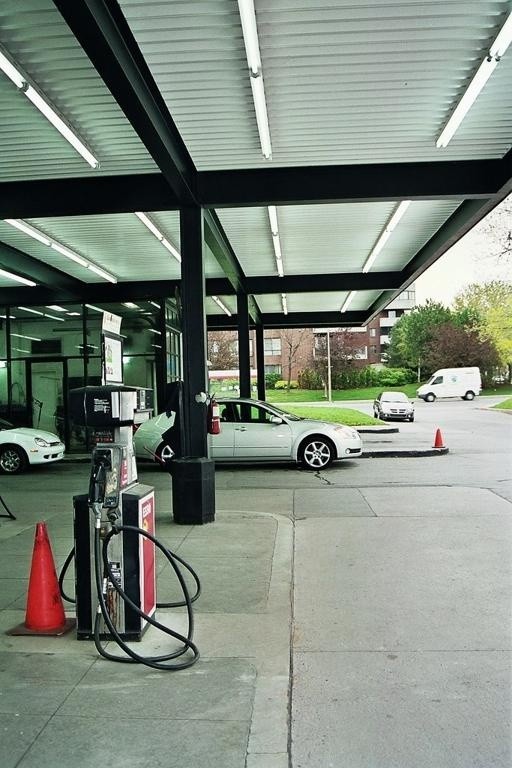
[171,455,215,525]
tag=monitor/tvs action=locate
[30,338,63,356]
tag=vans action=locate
[416,365,482,402]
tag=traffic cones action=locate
[6,520,80,636]
[432,428,446,449]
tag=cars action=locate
[131,396,363,474]
[0,418,67,474]
[373,391,414,423]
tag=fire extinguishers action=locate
[207,393,220,434]
